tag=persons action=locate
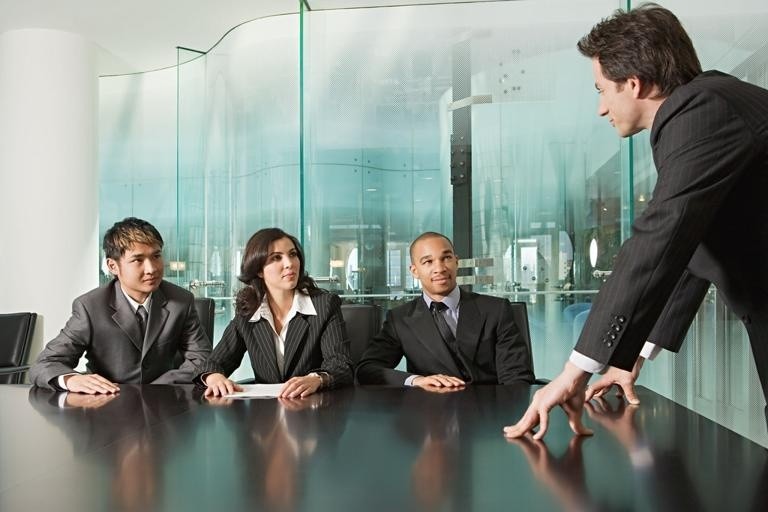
[348,230,538,391]
[27,215,215,397]
[24,382,198,511]
[501,1,768,445]
[371,389,531,510]
[502,394,767,510]
[190,227,354,400]
[200,395,354,512]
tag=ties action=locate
[137,306,147,340]
[430,301,475,385]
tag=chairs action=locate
[0,312,38,384]
[192,297,215,344]
[508,301,532,353]
[338,304,381,358]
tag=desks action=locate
[0,382,768,512]
